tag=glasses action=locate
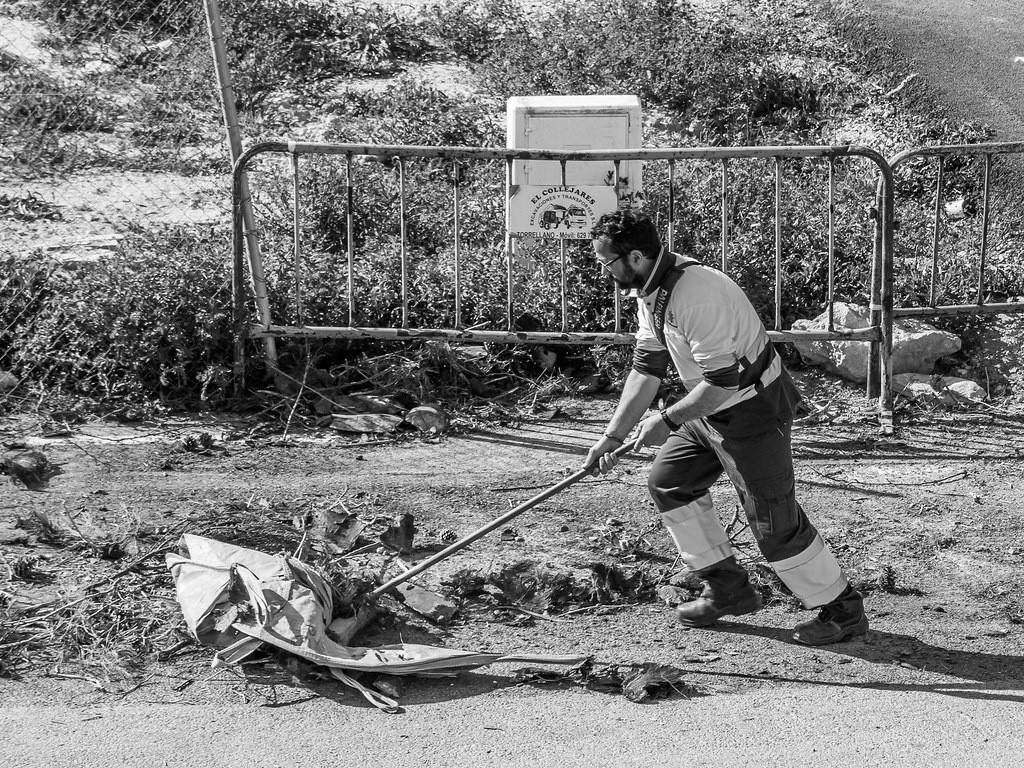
[598,251,645,272]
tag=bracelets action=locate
[657,397,682,432]
[604,430,623,447]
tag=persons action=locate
[581,207,870,647]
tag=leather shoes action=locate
[792,611,868,645]
[677,582,760,625]
